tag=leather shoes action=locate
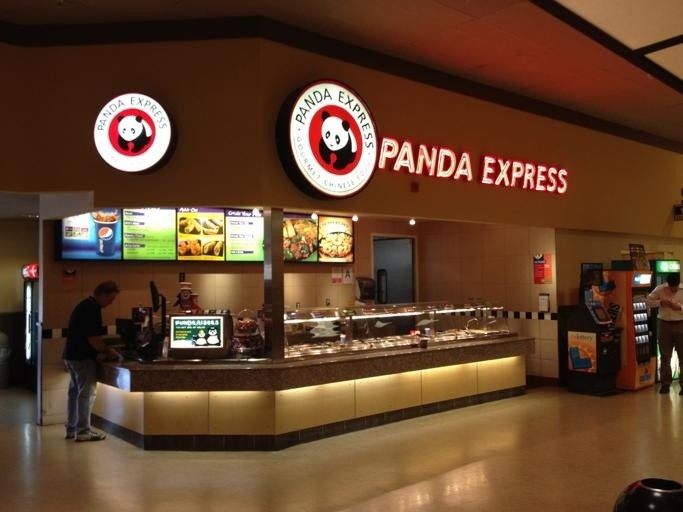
[658,383,669,394]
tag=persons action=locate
[645,272,683,395]
[63,280,121,441]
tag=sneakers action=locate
[76,430,107,442]
[66,430,78,439]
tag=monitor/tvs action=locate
[169,313,233,359]
[150,281,166,331]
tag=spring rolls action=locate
[203,241,223,255]
[180,219,201,236]
[203,220,223,235]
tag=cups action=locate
[179,282,200,310]
[340,334,346,343]
[94,219,118,256]
[424,328,430,336]
